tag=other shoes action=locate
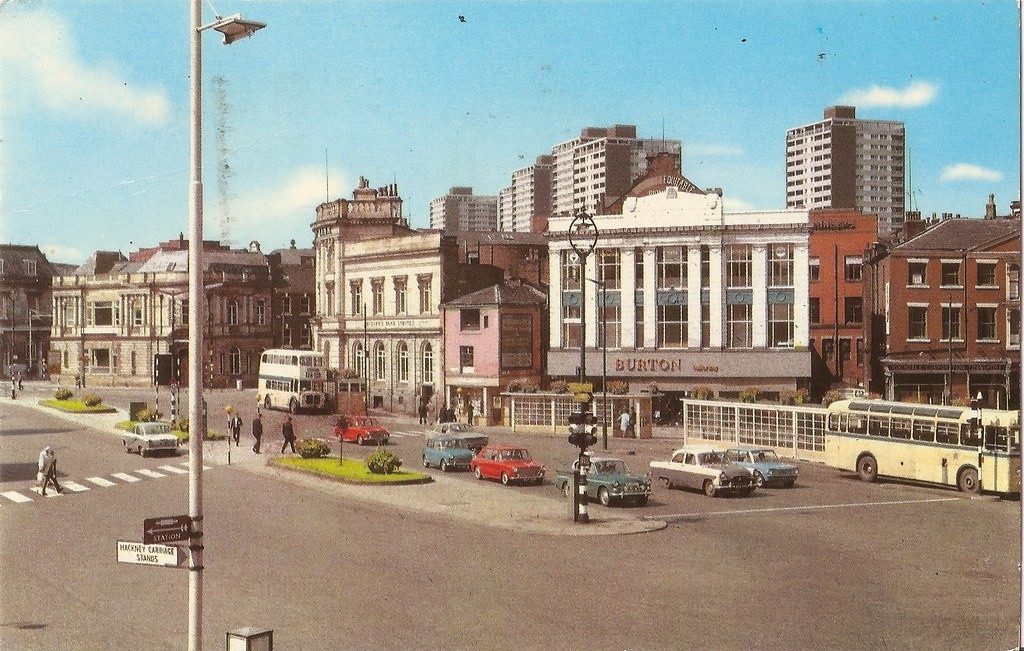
[42,492,47,496]
[58,487,63,493]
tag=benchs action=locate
[853,427,958,444]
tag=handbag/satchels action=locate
[37,472,44,479]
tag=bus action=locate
[257,347,327,415]
[825,400,1022,498]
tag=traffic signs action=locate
[116,539,187,569]
[143,514,192,545]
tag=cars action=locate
[471,444,545,485]
[334,415,390,445]
[723,447,799,488]
[421,436,475,471]
[555,456,651,506]
[650,449,754,497]
[122,423,179,457]
[432,423,489,452]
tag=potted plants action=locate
[53,388,73,401]
[362,445,402,475]
[81,394,103,407]
[136,408,163,423]
[293,436,332,459]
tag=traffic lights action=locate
[584,414,597,445]
[568,413,583,446]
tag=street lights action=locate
[121,282,224,429]
[188,11,266,651]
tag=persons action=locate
[604,466,616,475]
[253,414,264,454]
[282,417,297,454]
[617,407,636,438]
[37,446,65,496]
[229,412,243,446]
[418,400,475,425]
[513,450,521,461]
[711,452,767,463]
[41,366,49,381]
[17,372,23,390]
